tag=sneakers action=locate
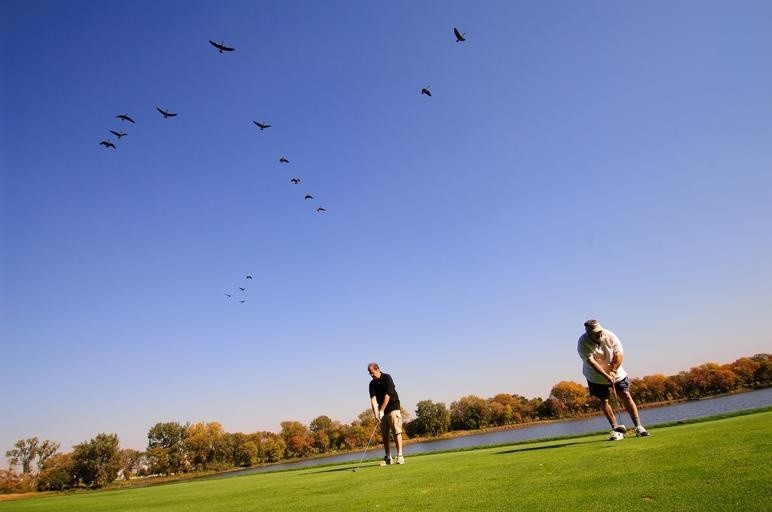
[635,425,650,437]
[609,431,624,441]
[395,455,405,464]
[379,457,394,466]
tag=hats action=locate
[584,319,604,333]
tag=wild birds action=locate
[253,120,271,131]
[208,39,236,54]
[280,156,288,164]
[116,113,136,123]
[421,85,432,97]
[156,107,177,119]
[290,178,300,184]
[99,141,116,149]
[109,129,127,138]
[453,27,467,44]
[317,208,326,212]
[304,194,313,200]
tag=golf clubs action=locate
[613,384,626,434]
[352,421,378,472]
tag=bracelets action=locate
[609,369,617,374]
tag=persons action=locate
[577,319,650,440]
[368,362,406,466]
